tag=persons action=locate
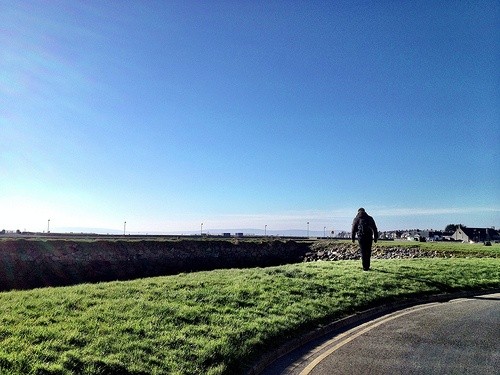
[352,208,378,272]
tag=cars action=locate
[484,241,491,246]
